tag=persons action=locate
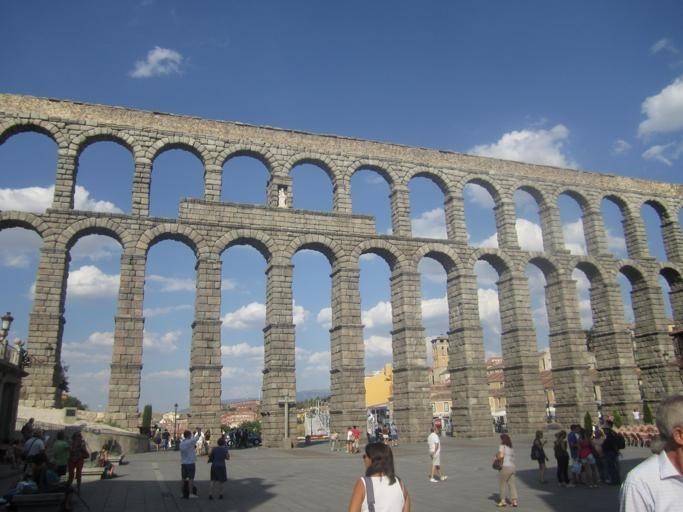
[8,416,116,495]
[494,433,518,508]
[529,414,624,488]
[348,442,411,512]
[325,420,402,452]
[207,438,229,500]
[618,395,682,512]
[151,423,255,452]
[426,427,448,482]
[179,427,201,498]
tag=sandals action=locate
[512,501,517,507]
[496,501,507,507]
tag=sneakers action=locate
[440,476,448,480]
[540,476,611,488]
[430,478,438,482]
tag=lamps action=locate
[24,342,54,369]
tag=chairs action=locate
[616,423,660,448]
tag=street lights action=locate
[543,389,551,422]
[171,401,178,451]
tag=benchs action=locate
[10,491,66,511]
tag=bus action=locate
[376,408,389,433]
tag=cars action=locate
[317,428,327,436]
[224,431,261,447]
[305,411,316,418]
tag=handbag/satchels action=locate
[492,457,503,470]
[27,453,46,463]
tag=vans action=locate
[432,415,448,430]
